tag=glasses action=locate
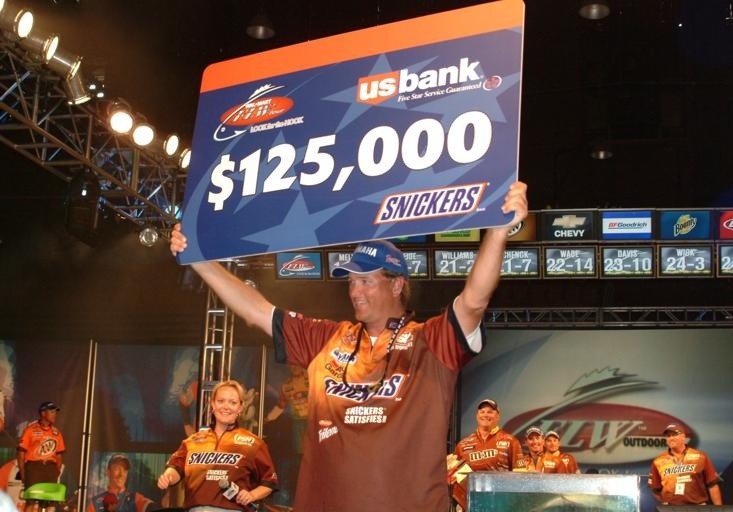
[343,346,391,394]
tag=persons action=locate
[157,381,280,512]
[521,428,557,472]
[169,182,529,512]
[17,402,66,511]
[546,431,580,473]
[86,455,155,512]
[646,424,722,505]
[446,398,521,511]
[266,356,308,443]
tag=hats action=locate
[332,240,409,279]
[39,401,61,411]
[545,431,560,440]
[526,428,544,439]
[478,399,500,414]
[663,424,683,434]
[108,454,131,468]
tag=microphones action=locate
[218,478,257,510]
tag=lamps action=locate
[45,47,84,80]
[137,221,161,247]
[131,114,157,149]
[61,71,93,107]
[18,26,61,64]
[155,129,181,157]
[0,1,37,41]
[106,98,134,136]
[169,145,192,170]
